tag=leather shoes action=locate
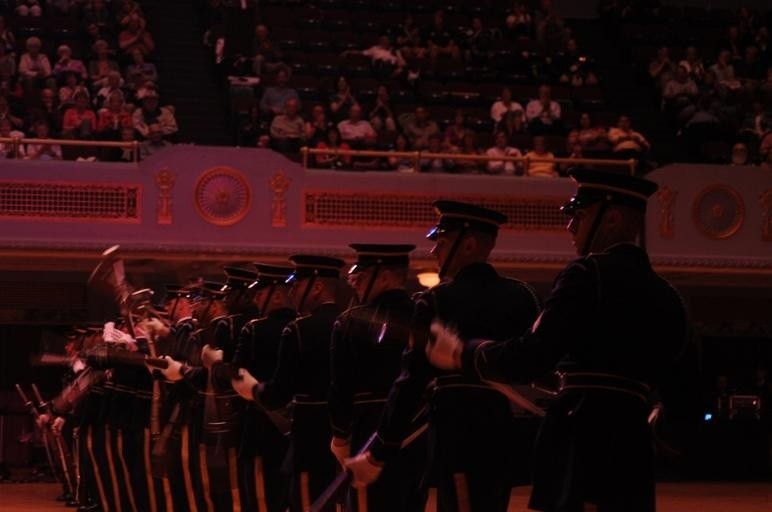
[76,500,101,512]
[56,491,82,507]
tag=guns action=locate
[107,350,200,372]
[309,377,437,512]
[366,311,553,417]
[30,383,80,501]
[15,382,67,484]
[36,246,295,467]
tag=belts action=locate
[352,391,388,406]
[291,394,328,405]
[558,373,650,401]
[433,374,498,392]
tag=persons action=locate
[34,166,772,510]
[1,1,771,177]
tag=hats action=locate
[161,281,223,300]
[346,243,417,275]
[220,266,258,292]
[424,200,509,240]
[63,304,169,344]
[284,254,347,285]
[559,168,660,214]
[247,262,297,291]
